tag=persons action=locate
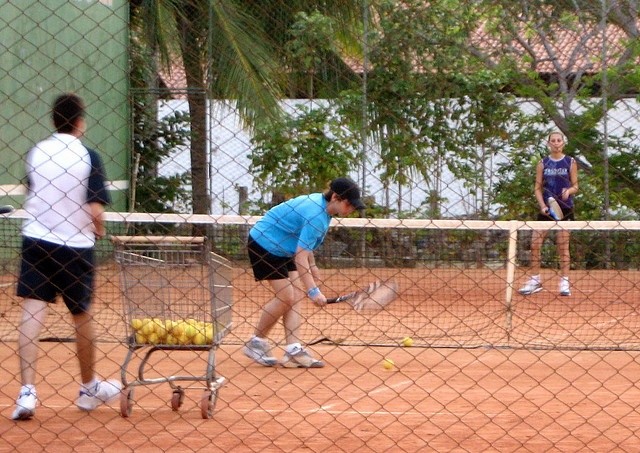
[515,132,579,296]
[10,93,124,419]
[241,177,368,367]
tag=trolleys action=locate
[110,235,234,419]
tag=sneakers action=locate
[558,282,570,296]
[280,348,324,367]
[244,340,277,367]
[76,378,122,410]
[518,279,542,295]
[13,393,37,419]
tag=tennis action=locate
[382,359,393,369]
[403,337,413,347]
[130,318,224,344]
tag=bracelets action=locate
[307,287,319,299]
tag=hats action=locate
[330,178,366,209]
